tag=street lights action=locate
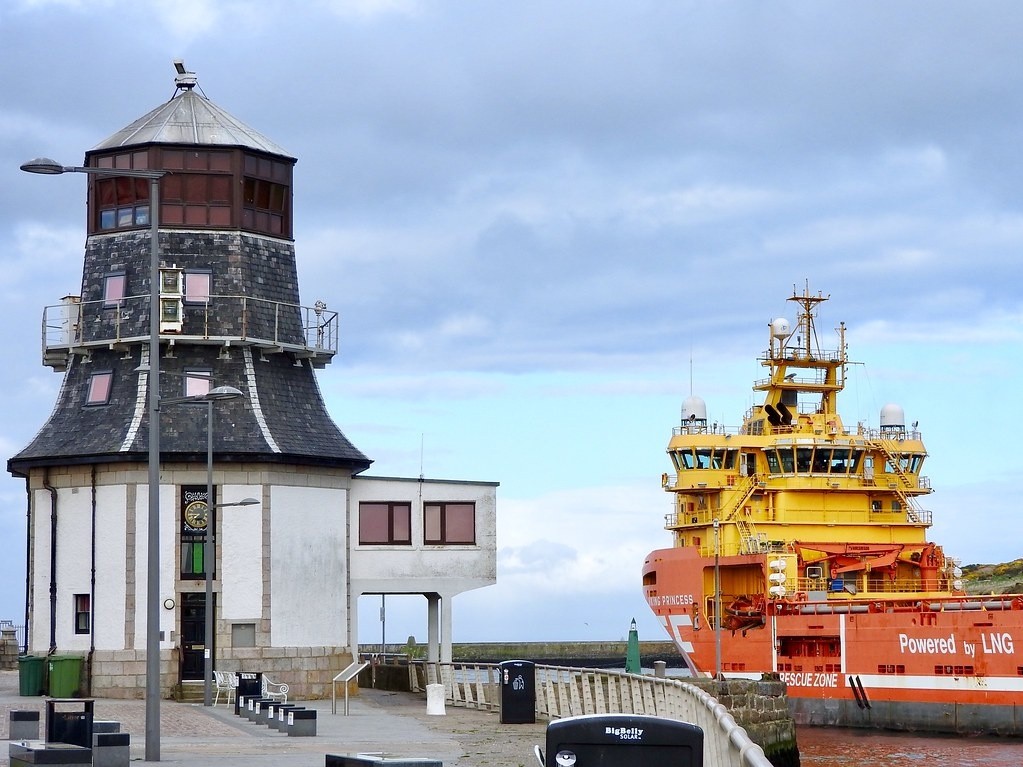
[130,364,261,704]
[20,158,243,762]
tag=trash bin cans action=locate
[44,697,94,747]
[497,660,536,724]
[534,712,705,767]
[234,671,264,716]
[48,654,82,698]
[16,654,46,696]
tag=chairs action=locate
[213,671,289,709]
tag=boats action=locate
[640,279,1023,740]
